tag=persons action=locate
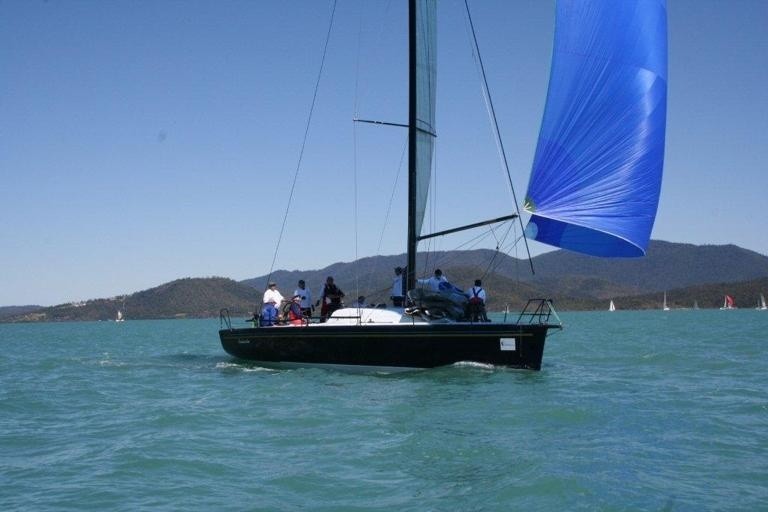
[260,266,491,326]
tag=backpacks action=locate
[469,288,483,311]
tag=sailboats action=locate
[663,291,670,311]
[719,294,737,310]
[754,292,768,311]
[218,1,670,371]
[116,310,125,322]
[608,299,615,312]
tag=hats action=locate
[393,266,401,271]
[268,282,276,286]
